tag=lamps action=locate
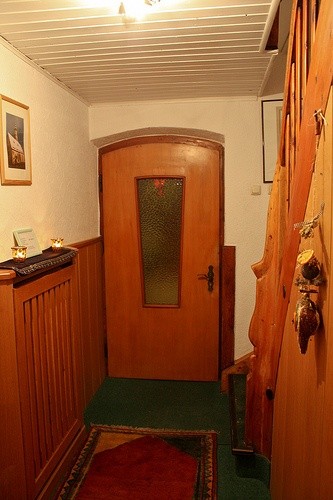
[51,239,63,253]
[11,246,28,264]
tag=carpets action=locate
[57,424,218,500]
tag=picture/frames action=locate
[261,99,283,183]
[0,93,32,186]
